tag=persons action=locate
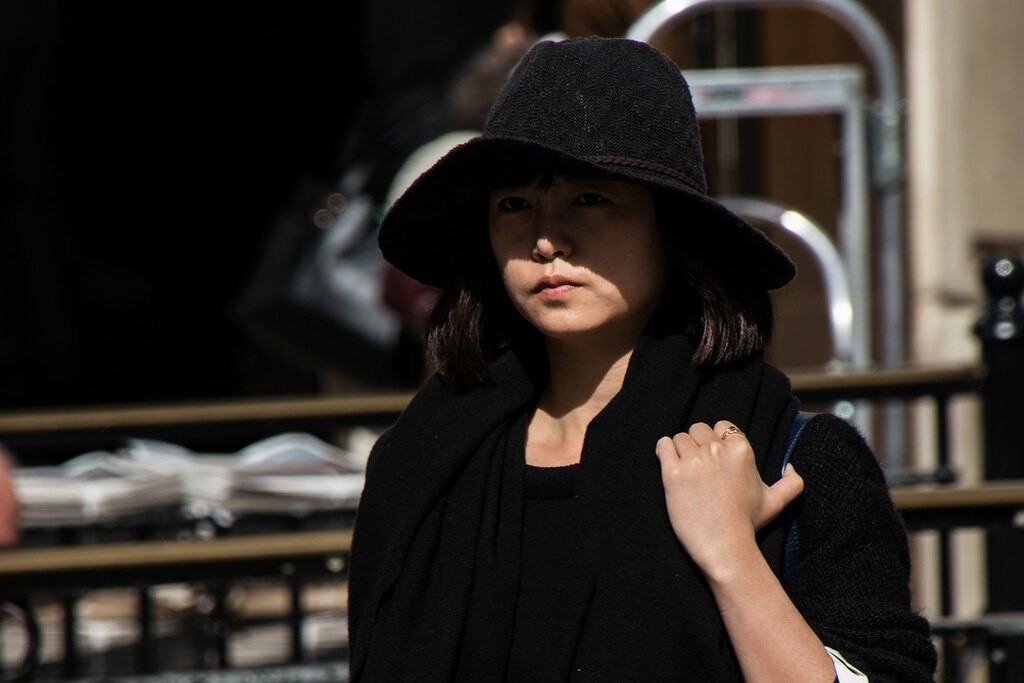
[346,36,940,683]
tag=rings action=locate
[721,426,746,440]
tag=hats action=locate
[377,37,797,289]
